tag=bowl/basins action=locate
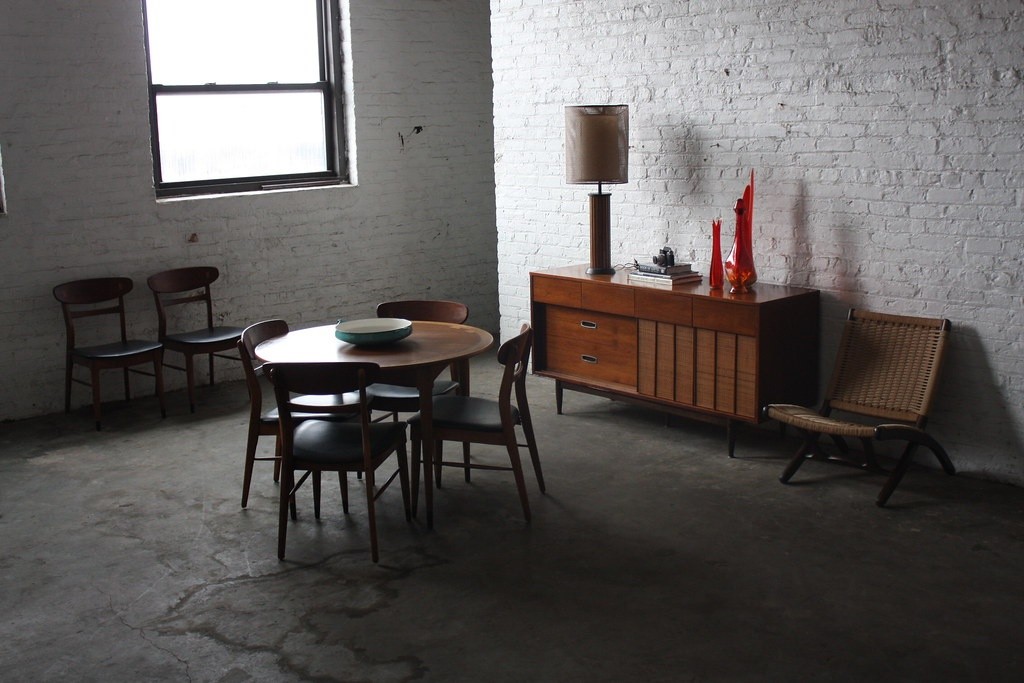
[334,317,413,345]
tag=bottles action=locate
[709,219,725,291]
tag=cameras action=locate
[653,247,675,268]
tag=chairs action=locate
[147,266,251,414]
[52,277,167,431]
[761,308,955,507]
[237,300,546,564]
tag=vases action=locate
[708,220,724,287]
[724,168,758,295]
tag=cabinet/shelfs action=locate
[529,263,820,458]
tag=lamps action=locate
[565,105,629,274]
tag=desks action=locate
[255,322,493,529]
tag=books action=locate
[627,262,703,285]
